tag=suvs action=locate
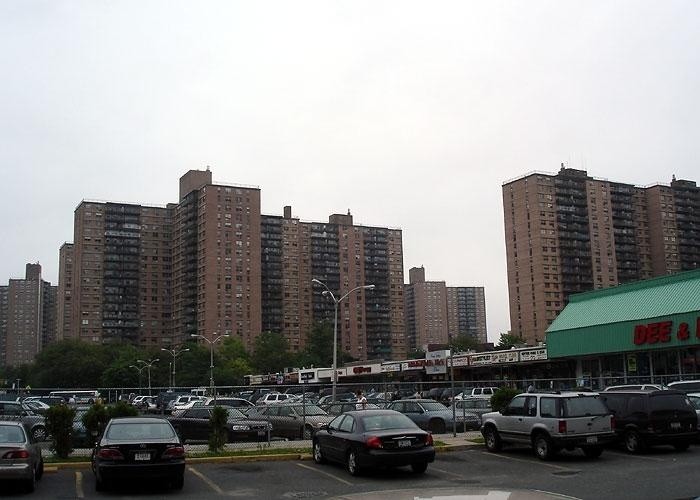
[598,381,700,455]
[479,387,618,458]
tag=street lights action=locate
[129,365,147,396]
[162,345,190,393]
[191,332,230,395]
[137,359,160,396]
[311,278,376,405]
[15,378,23,394]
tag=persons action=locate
[415,391,421,399]
[68,397,75,406]
[355,391,368,410]
[88,396,95,404]
[528,382,535,393]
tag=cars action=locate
[92,415,186,493]
[312,409,436,477]
[1,421,43,493]
